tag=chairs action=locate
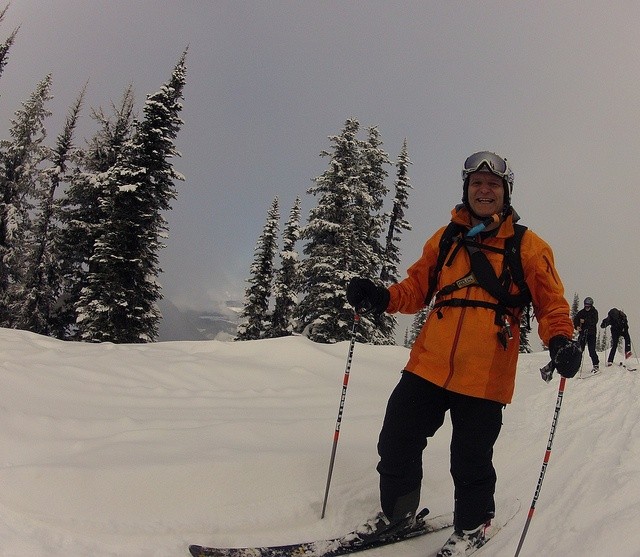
[608,362,612,366]
[437,521,488,557]
[356,508,417,540]
[592,365,600,373]
[625,351,632,360]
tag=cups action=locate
[463,151,514,184]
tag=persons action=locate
[600,307,633,367]
[346,151,582,555]
[573,296,599,373]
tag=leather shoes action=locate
[548,336,582,377]
[346,276,389,314]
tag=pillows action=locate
[584,297,594,304]
